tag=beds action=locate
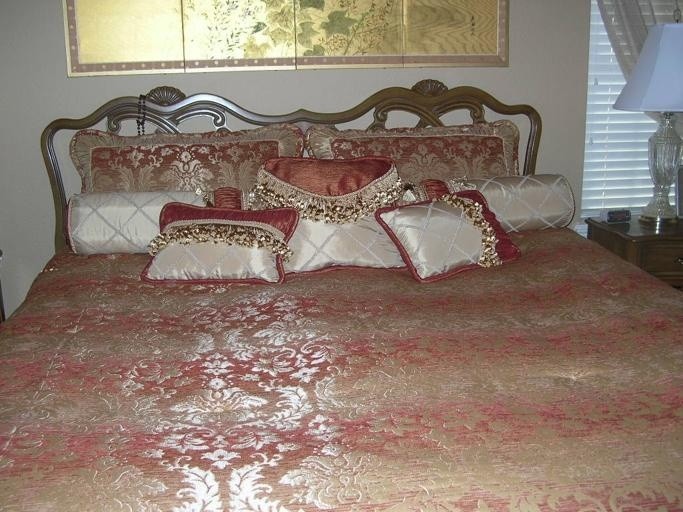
[0,79,683,512]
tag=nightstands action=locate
[583,216,683,292]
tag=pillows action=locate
[375,190,523,285]
[302,118,522,182]
[142,198,298,289]
[63,187,207,257]
[68,120,305,211]
[252,154,414,280]
[463,172,577,235]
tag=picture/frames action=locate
[62,1,511,80]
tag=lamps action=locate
[612,22,683,227]
